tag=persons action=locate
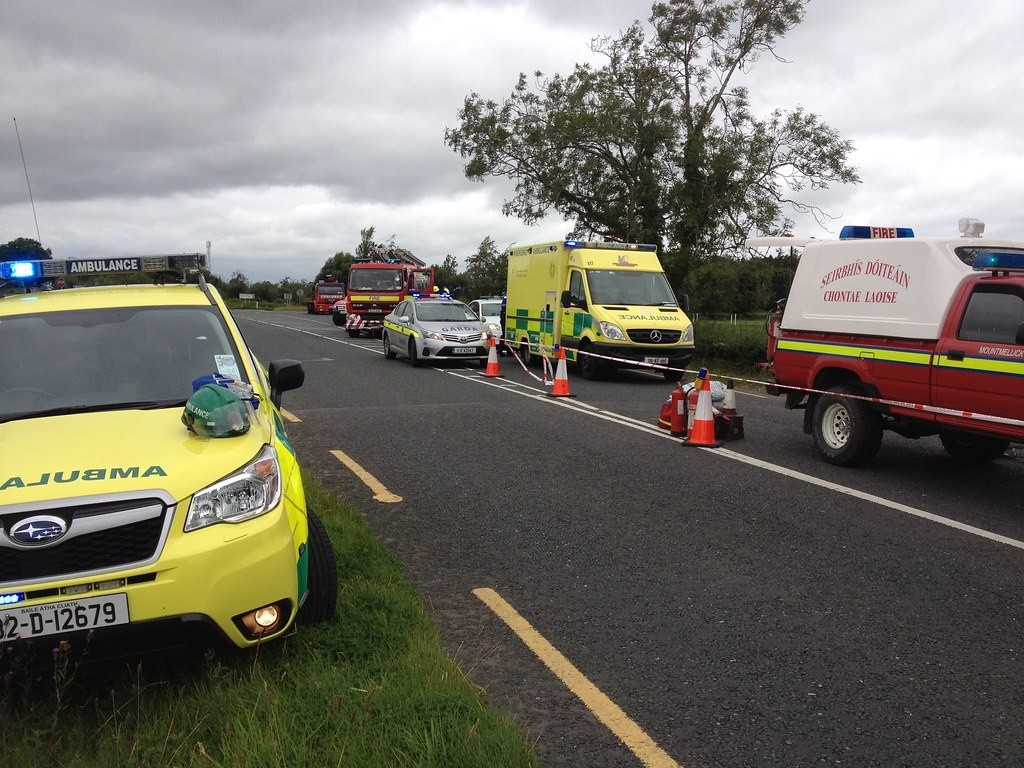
[496,296,516,358]
[433,285,450,295]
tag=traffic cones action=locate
[719,380,744,417]
[480,337,506,378]
[545,347,577,397]
[683,374,722,448]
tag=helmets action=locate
[444,288,450,294]
[433,286,439,292]
[182,384,251,438]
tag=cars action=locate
[1,255,338,651]
[381,294,494,367]
[468,295,506,343]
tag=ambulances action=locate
[506,241,695,382]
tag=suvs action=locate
[332,297,346,326]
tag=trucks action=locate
[304,281,346,315]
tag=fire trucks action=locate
[746,219,1024,468]
[346,249,435,338]
